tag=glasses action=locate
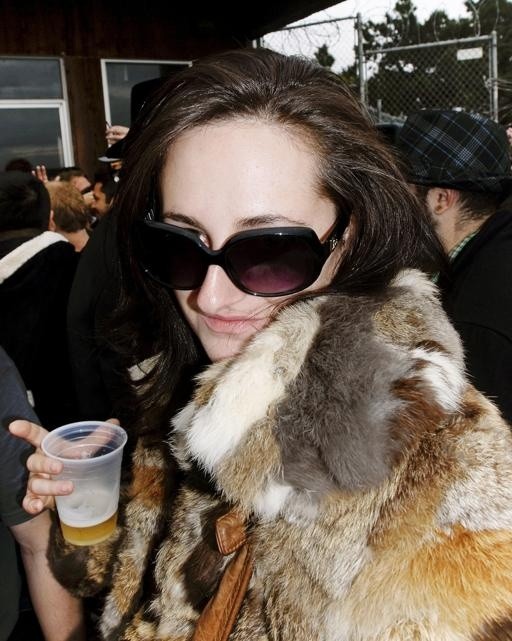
[131,208,352,297]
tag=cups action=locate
[41,421,129,547]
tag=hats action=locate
[371,109,511,192]
[105,77,168,158]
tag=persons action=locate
[369,98,510,429]
[8,45,510,641]
[0,136,168,641]
